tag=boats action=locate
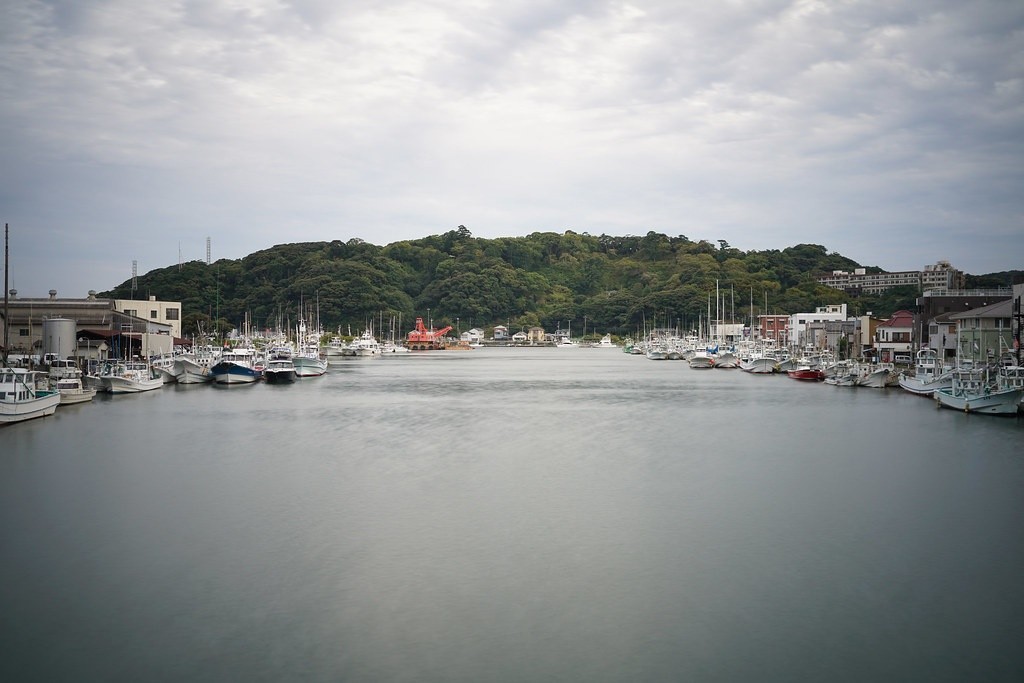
[470,343,485,349]
[557,337,579,348]
[591,333,616,348]
[0,346,261,424]
[778,316,1024,416]
[264,346,296,384]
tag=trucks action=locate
[7,352,60,368]
[6,358,40,368]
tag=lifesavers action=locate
[413,345,418,350]
[420,345,425,350]
[429,345,433,349]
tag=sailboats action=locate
[624,280,779,375]
[293,289,411,376]
[194,303,294,347]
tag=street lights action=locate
[79,337,89,374]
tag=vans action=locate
[895,355,913,365]
[49,360,83,378]
[86,359,106,373]
